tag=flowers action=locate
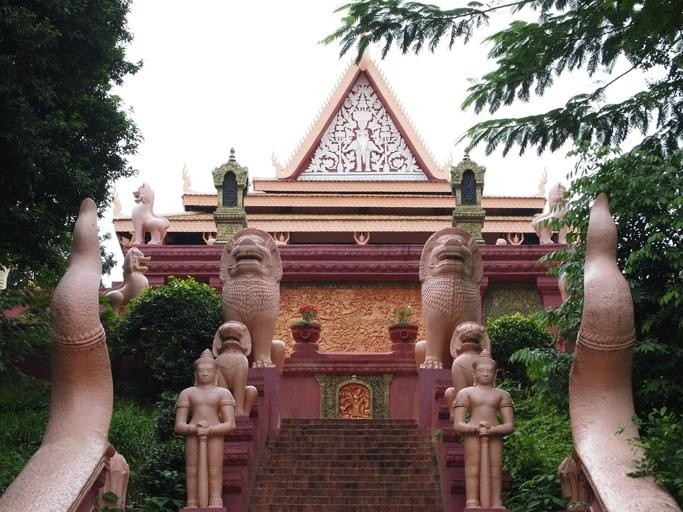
[299,304,318,322]
[392,304,415,321]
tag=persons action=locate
[174,349,235,508]
[453,349,514,507]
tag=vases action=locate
[390,325,418,342]
[291,323,321,342]
